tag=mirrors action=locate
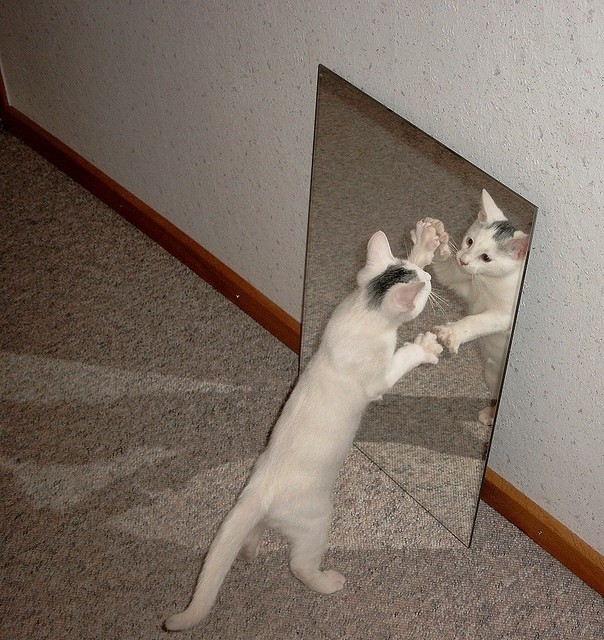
[297,64,537,549]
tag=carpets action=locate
[0,127,604,639]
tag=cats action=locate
[423,189,531,426]
[163,218,445,632]
